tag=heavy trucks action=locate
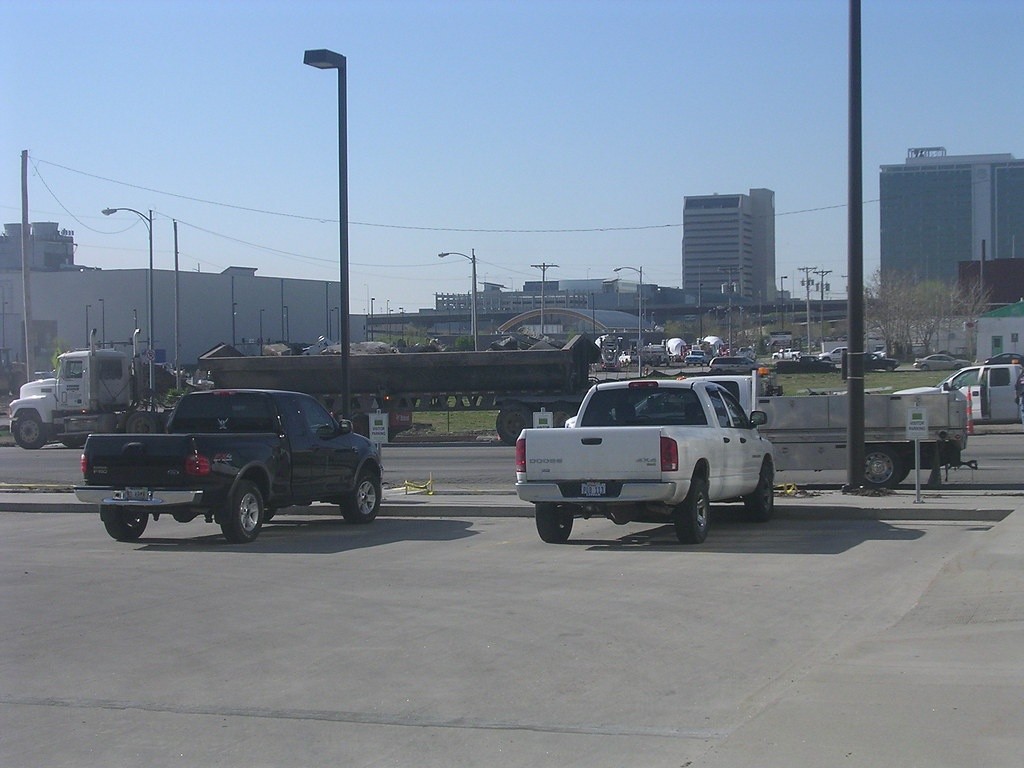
[9,333,604,450]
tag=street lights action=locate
[386,300,390,336]
[99,299,105,349]
[133,308,137,329]
[326,282,332,337]
[613,266,643,340]
[370,298,376,341]
[232,303,238,345]
[389,310,394,342]
[399,308,404,349]
[284,305,289,343]
[699,283,705,338]
[259,308,265,341]
[335,307,340,340]
[85,305,92,350]
[781,276,788,331]
[101,208,156,394]
[591,293,596,336]
[303,49,350,421]
[364,284,369,314]
[438,248,475,336]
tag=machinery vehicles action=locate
[591,336,758,372]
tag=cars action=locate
[984,352,1024,366]
[865,350,902,371]
[796,332,880,343]
[913,353,973,371]
[819,347,848,364]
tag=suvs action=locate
[709,355,767,375]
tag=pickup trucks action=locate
[891,363,1024,426]
[72,388,385,544]
[772,349,802,361]
[775,355,842,374]
[514,379,778,546]
[685,350,711,366]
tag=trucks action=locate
[629,369,980,492]
